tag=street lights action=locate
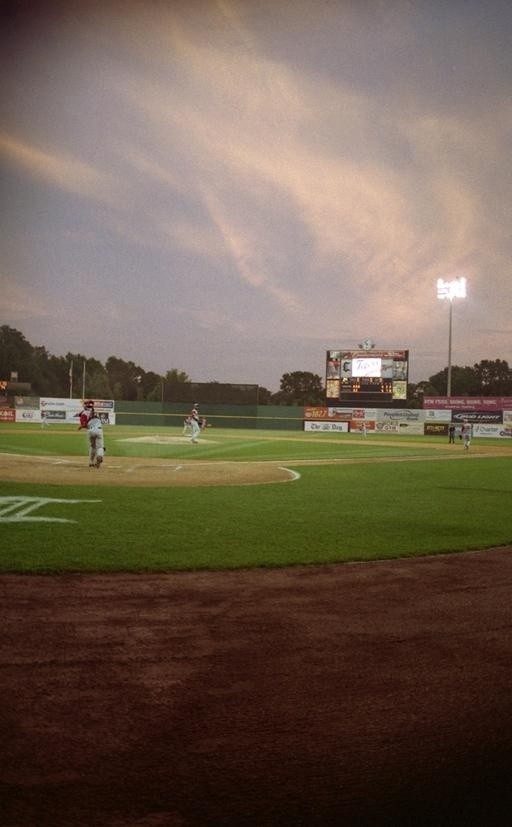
[436,276,468,396]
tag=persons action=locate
[182,403,211,443]
[449,424,455,443]
[87,416,104,468]
[361,424,367,439]
[461,419,473,450]
[41,410,50,430]
